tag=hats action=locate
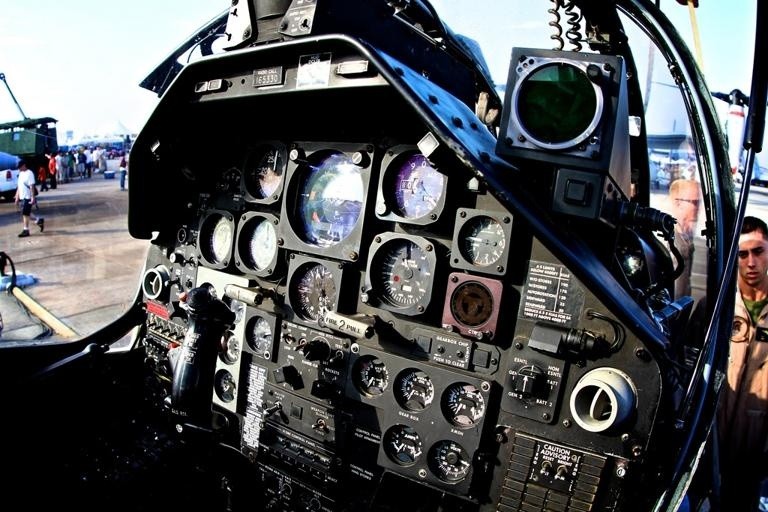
[18,160,24,168]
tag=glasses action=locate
[677,197,702,205]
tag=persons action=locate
[14,160,45,238]
[666,178,701,302]
[727,213,767,411]
[37,145,127,194]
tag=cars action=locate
[0,149,21,200]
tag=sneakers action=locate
[35,218,44,232]
[18,229,29,237]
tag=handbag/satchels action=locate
[34,188,38,195]
